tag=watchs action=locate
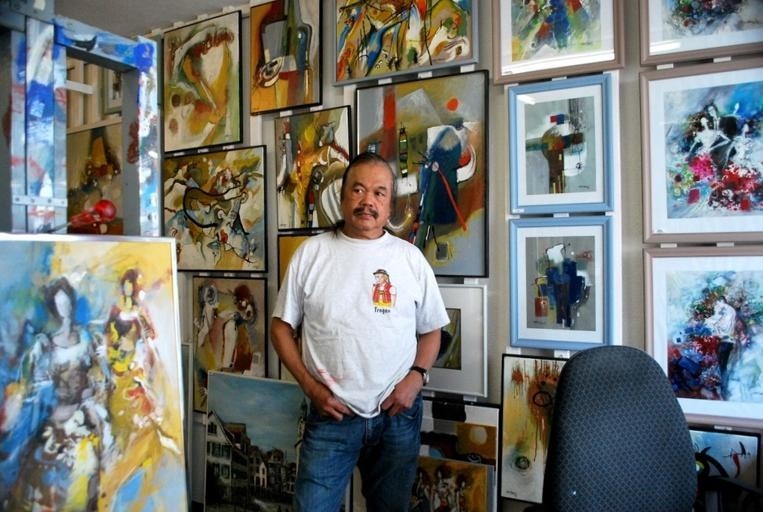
[410,366,430,386]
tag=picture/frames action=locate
[497,0,625,83]
[508,73,615,215]
[640,247,763,434]
[638,0,763,67]
[0,234,189,512]
[98,66,123,115]
[510,218,613,351]
[639,62,763,245]
[499,353,570,507]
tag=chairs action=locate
[540,345,762,512]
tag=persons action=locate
[685,101,757,177]
[410,465,468,512]
[702,295,736,399]
[2,277,106,512]
[269,152,451,512]
[104,269,178,512]
